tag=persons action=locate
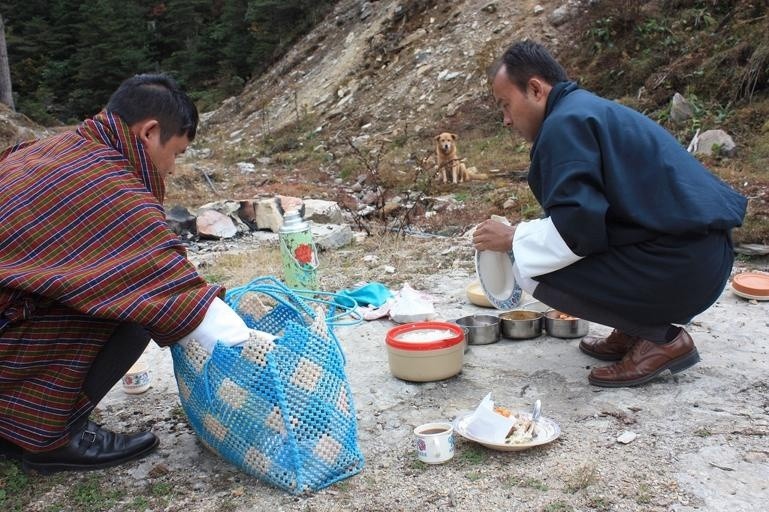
[470,37,749,389]
[0,69,252,472]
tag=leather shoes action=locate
[589,327,699,387]
[22,420,160,471]
[579,328,640,361]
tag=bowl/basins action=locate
[544,309,589,338]
[456,315,501,345]
[385,322,464,382]
[466,279,494,307]
[499,311,543,340]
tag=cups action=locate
[122,363,151,394]
[414,423,454,465]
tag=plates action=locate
[474,249,523,310]
[730,272,769,301]
[456,410,561,452]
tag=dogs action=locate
[433,132,471,185]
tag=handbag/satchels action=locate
[169,276,365,497]
[334,283,394,318]
